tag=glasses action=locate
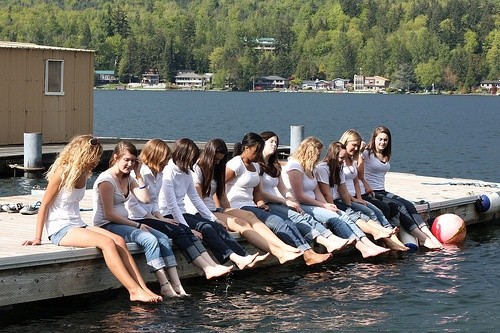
[89,139,101,147]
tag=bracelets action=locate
[136,222,142,229]
[33,237,42,241]
[137,183,149,190]
[135,173,143,179]
[366,188,374,196]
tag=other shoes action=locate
[79,208,94,212]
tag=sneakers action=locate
[0,199,41,215]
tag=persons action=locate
[253,131,357,253]
[22,134,164,303]
[90,140,190,297]
[313,141,398,241]
[280,135,390,259]
[157,138,271,272]
[189,136,306,264]
[357,125,445,252]
[216,131,333,267]
[336,128,412,254]
[125,137,235,280]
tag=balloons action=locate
[430,213,468,248]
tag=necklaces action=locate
[345,158,356,175]
